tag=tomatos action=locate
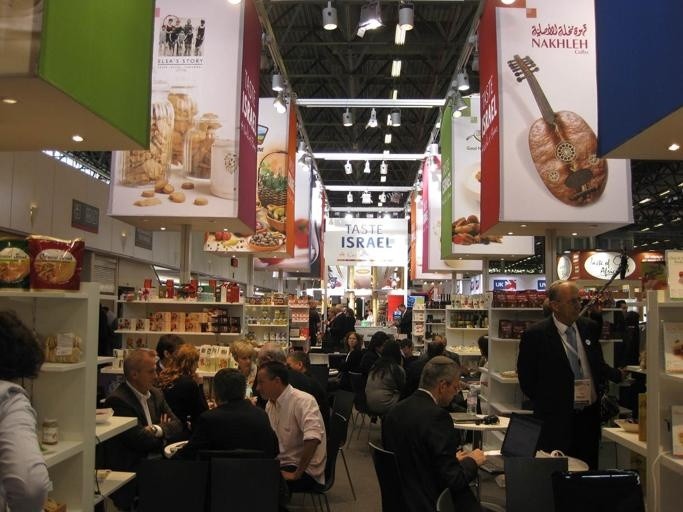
[295,218,310,249]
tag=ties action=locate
[566,327,585,411]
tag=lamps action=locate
[343,107,401,127]
[272,74,287,114]
[447,67,470,118]
[347,191,386,204]
[344,160,388,174]
[322,0,415,31]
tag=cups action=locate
[458,320,473,328]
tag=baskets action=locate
[258,151,289,206]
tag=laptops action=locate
[447,410,480,425]
[474,412,542,474]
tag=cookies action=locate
[121,91,221,208]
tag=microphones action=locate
[618,239,630,280]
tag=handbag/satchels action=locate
[554,469,645,512]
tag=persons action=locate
[340,331,446,416]
[308,298,357,353]
[614,299,628,315]
[384,355,483,512]
[160,17,205,56]
[0,312,51,512]
[516,280,622,472]
[367,309,374,327]
[477,334,488,414]
[103,337,328,512]
[398,304,412,339]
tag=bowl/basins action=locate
[614,419,639,432]
[95,407,115,423]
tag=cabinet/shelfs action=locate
[0,0,155,151]
[0,283,137,512]
[114,299,309,407]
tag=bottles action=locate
[426,325,430,339]
[440,293,451,309]
[41,416,59,445]
[118,79,235,200]
[220,282,240,303]
[466,385,478,417]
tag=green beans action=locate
[208,231,277,251]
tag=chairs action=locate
[302,354,409,512]
[137,458,280,512]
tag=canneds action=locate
[42,417,58,445]
